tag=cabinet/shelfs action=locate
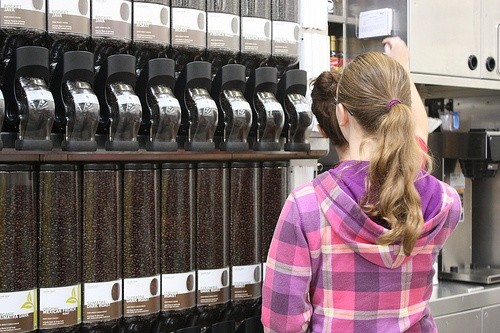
[406,1,500,93]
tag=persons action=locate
[261,50,461,333]
[312,36,429,179]
